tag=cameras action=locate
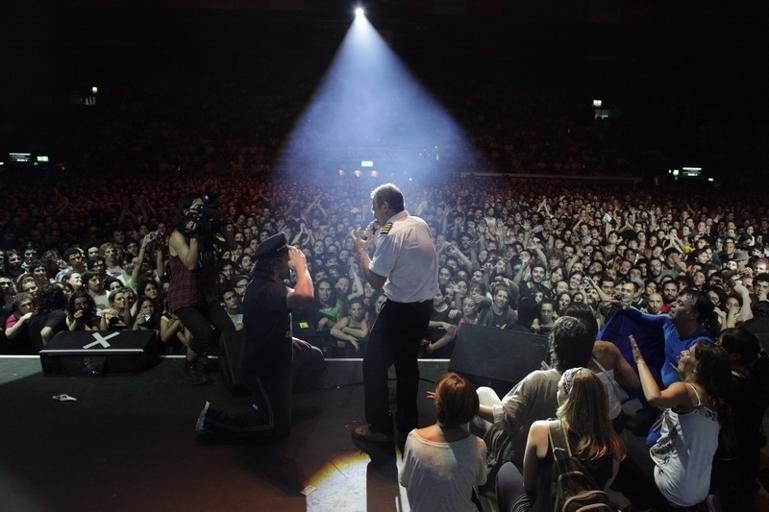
[144,314,151,322]
[153,228,165,239]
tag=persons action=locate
[350,183,439,442]
[0,80,401,358]
[195,232,314,445]
[401,78,769,512]
[166,193,235,385]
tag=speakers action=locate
[38,330,159,375]
[216,326,248,394]
[448,322,548,400]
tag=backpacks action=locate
[548,420,620,511]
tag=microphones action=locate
[359,218,380,243]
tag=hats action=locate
[254,232,287,259]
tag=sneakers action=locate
[352,426,395,442]
[196,399,212,431]
[184,358,210,371]
